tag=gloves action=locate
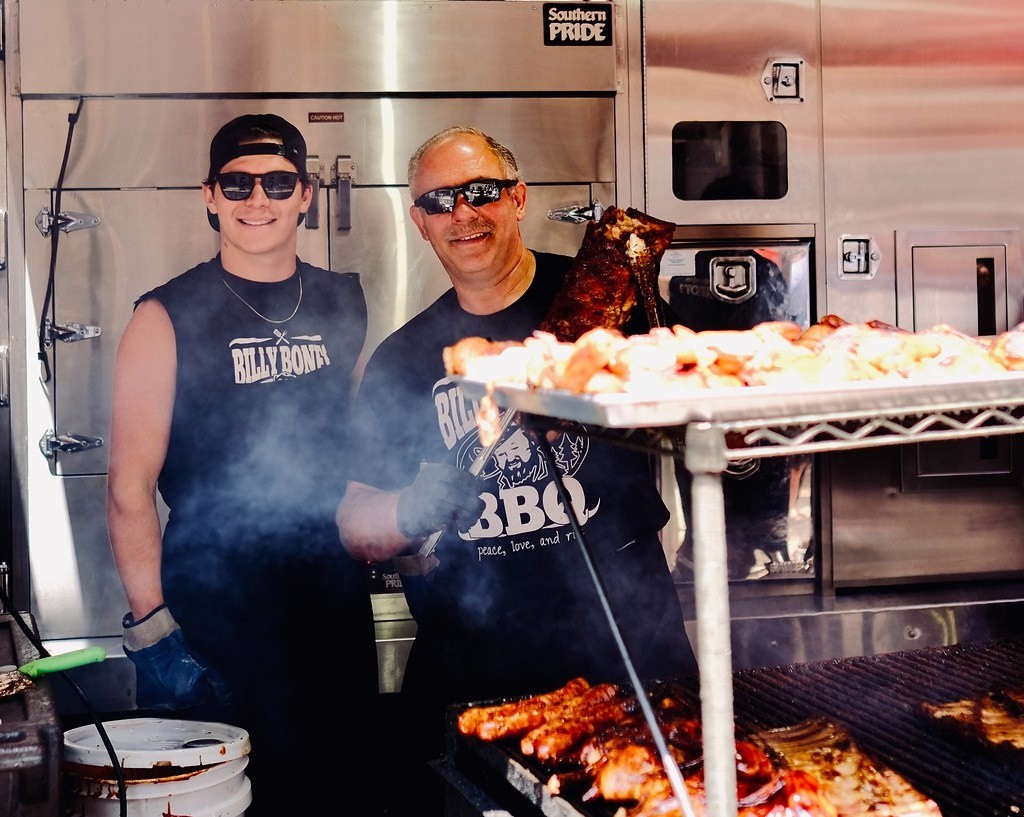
[122,604,233,712]
[398,464,486,538]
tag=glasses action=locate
[414,179,518,215]
[212,172,306,200]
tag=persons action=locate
[334,122,702,706]
[108,114,381,710]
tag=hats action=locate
[208,113,307,232]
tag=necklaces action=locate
[218,272,303,324]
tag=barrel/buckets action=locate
[61,717,253,817]
